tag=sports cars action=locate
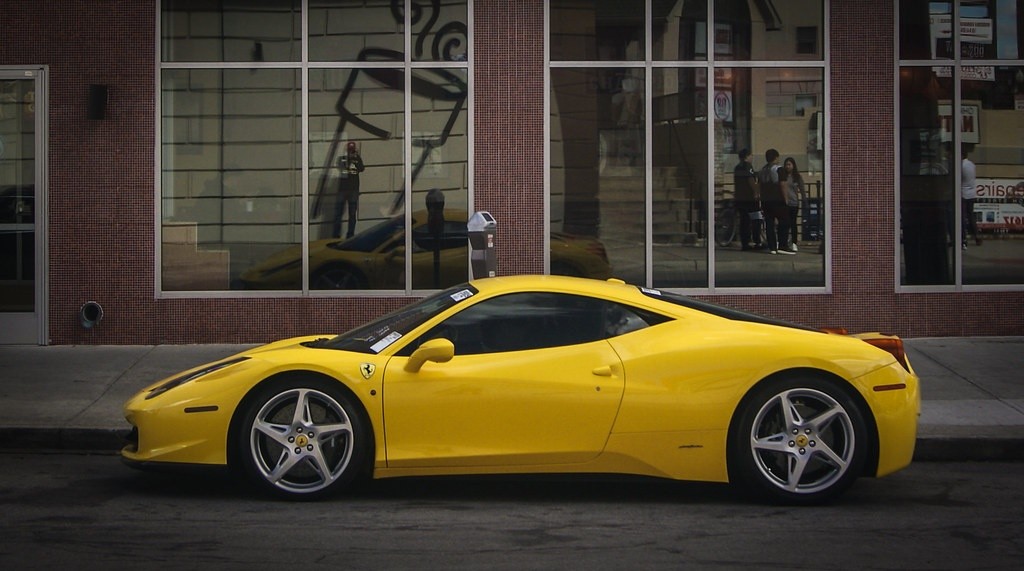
[121,273,924,510]
[232,209,614,290]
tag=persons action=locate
[333,142,364,238]
[734,149,808,255]
[961,150,982,248]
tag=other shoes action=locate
[976,238,983,246]
[962,240,968,250]
[792,242,798,252]
[769,249,796,256]
[741,243,766,251]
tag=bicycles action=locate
[714,198,779,248]
